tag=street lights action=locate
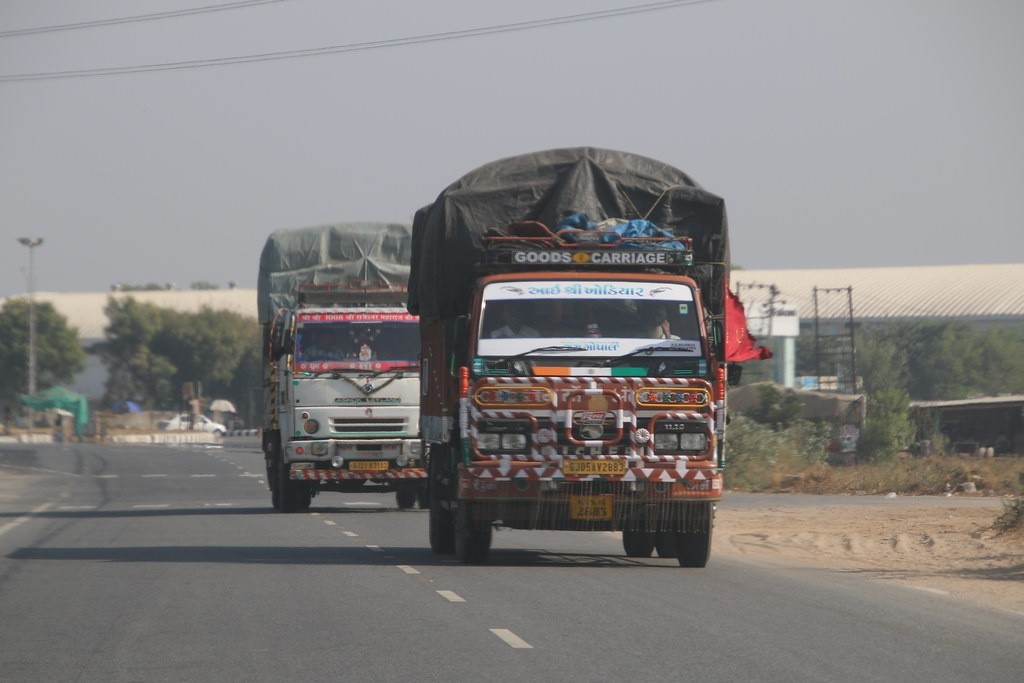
[18,237,43,434]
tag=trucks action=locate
[257,223,428,512]
[408,146,741,566]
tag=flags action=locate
[723,287,773,361]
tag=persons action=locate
[643,308,680,339]
[491,303,540,338]
[303,331,342,361]
